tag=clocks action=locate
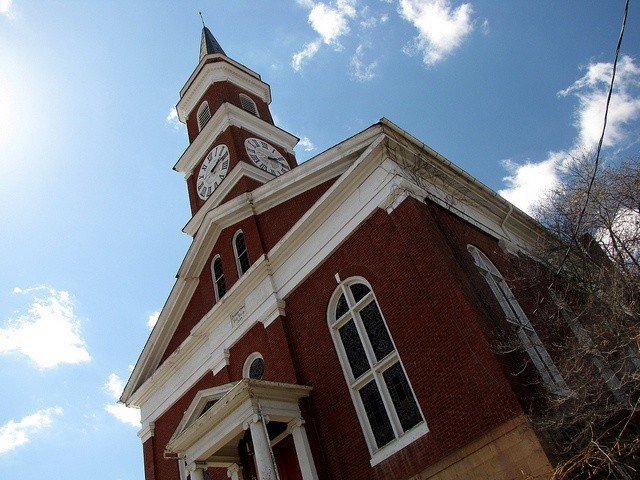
[244,137,292,176]
[196,144,230,201]
